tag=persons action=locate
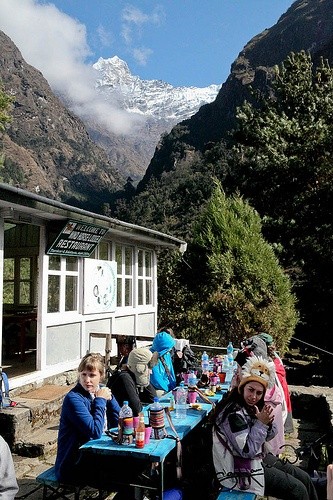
[107,331,320,500]
[0,436,19,500]
[55,353,150,500]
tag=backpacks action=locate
[0,370,10,409]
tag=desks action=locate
[17,311,37,362]
[78,363,232,500]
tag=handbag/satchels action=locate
[178,344,211,388]
[233,455,252,491]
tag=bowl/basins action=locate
[107,427,135,444]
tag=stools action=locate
[216,486,257,500]
[36,466,82,500]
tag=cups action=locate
[184,373,189,384]
[209,355,238,375]
[133,417,137,439]
[210,376,218,385]
[145,424,152,444]
[188,391,198,404]
[173,388,177,402]
[218,372,227,384]
[194,369,197,376]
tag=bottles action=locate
[119,401,133,445]
[189,371,197,392]
[227,341,234,355]
[136,417,145,448]
[228,353,233,366]
[150,397,165,439]
[176,383,186,419]
[222,355,229,372]
[170,395,175,410]
[201,351,209,371]
[209,358,215,372]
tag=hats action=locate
[234,333,274,371]
[237,351,276,395]
[127,348,158,393]
